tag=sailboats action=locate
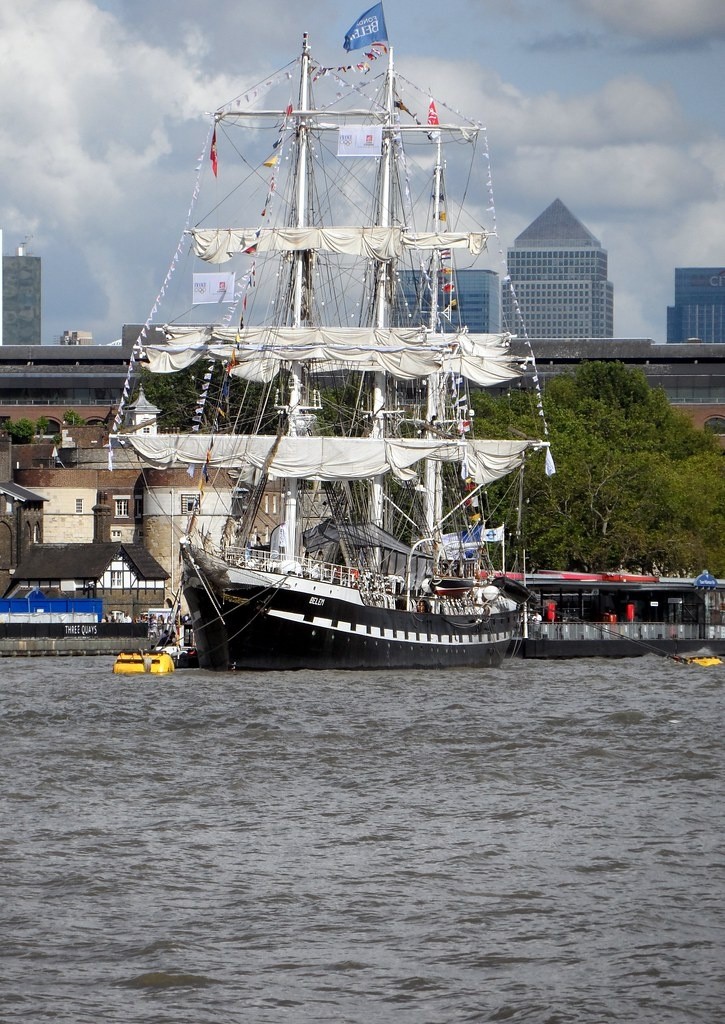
[90,2,559,675]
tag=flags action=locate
[112,348,135,432]
[211,228,262,349]
[306,43,387,82]
[193,271,235,303]
[193,350,239,481]
[343,1,388,52]
[429,101,439,126]
[134,235,186,344]
[545,448,556,478]
[262,104,292,217]
[212,130,218,177]
[438,527,482,561]
[186,481,205,534]
[336,124,382,157]
[450,373,482,525]
[432,193,459,311]
[484,526,505,542]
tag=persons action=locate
[103,611,176,645]
[528,609,542,638]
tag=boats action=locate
[492,568,725,661]
